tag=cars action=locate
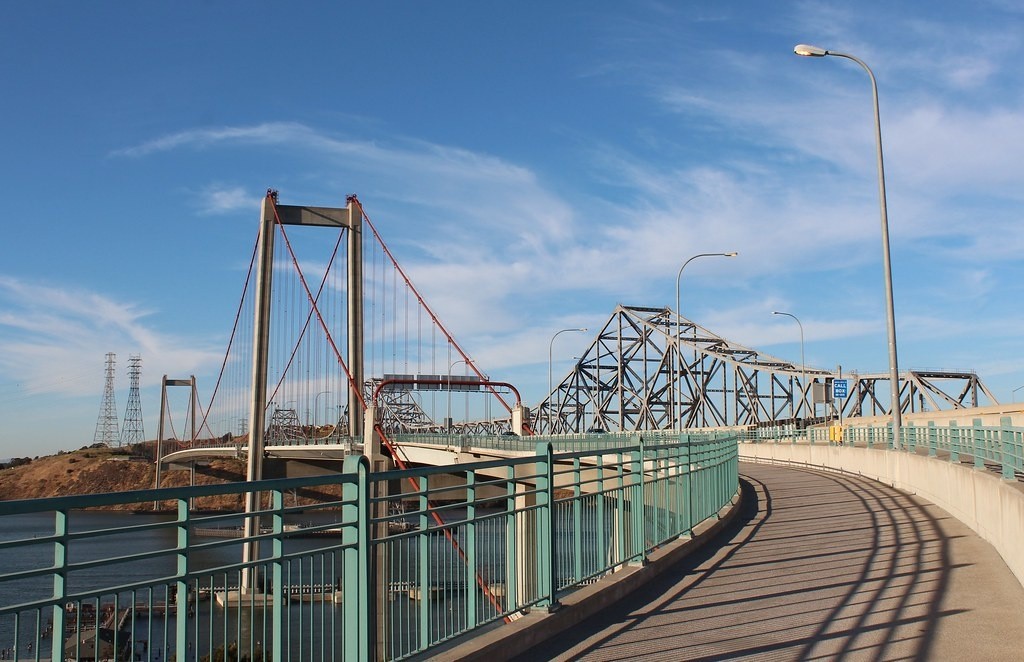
[502,432,520,441]
[586,429,610,440]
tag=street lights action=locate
[676,252,739,436]
[549,328,588,441]
[793,44,902,449]
[571,356,594,428]
[447,360,474,435]
[771,311,807,421]
[315,391,332,427]
